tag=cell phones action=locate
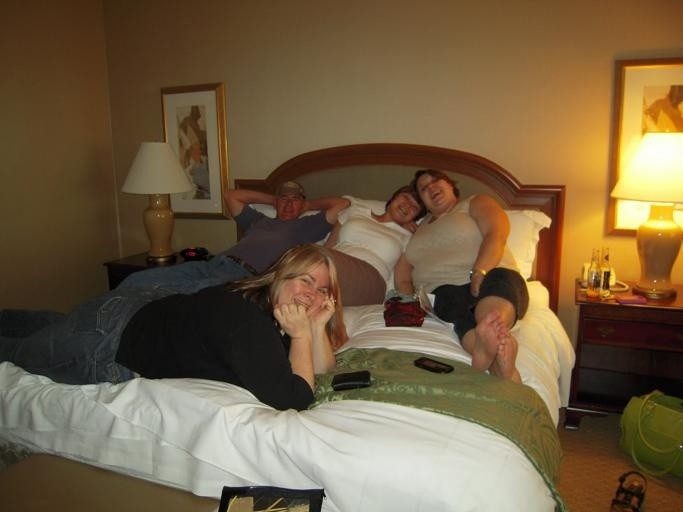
[414,356,454,374]
[617,294,647,304]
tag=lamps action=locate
[120,142,192,263]
[611,133,683,304]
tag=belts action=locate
[227,255,257,276]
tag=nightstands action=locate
[562,280,683,430]
[105,245,195,292]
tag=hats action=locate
[275,181,307,198]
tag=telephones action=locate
[583,261,616,288]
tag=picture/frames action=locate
[159,79,229,222]
[601,56,683,238]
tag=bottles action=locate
[601,248,611,298]
[585,246,600,299]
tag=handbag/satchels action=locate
[383,296,425,327]
[618,390,682,477]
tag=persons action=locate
[391,167,532,385]
[317,183,430,310]
[1,241,352,414]
[107,177,353,292]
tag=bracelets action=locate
[469,267,487,280]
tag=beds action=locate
[2,142,567,512]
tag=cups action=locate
[648,280,666,299]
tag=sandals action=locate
[608,472,646,512]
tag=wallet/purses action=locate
[331,370,371,391]
